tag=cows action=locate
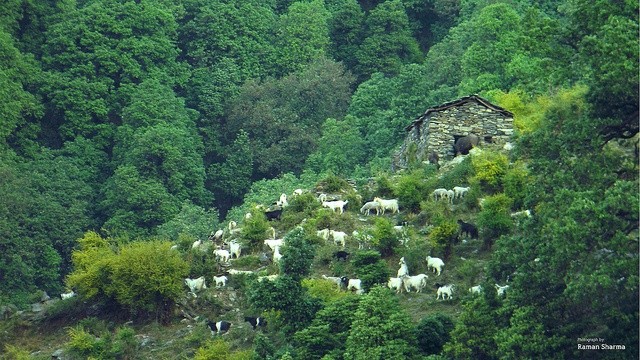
[450,133,480,158]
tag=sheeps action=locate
[213,276,228,286]
[374,196,400,215]
[351,231,375,250]
[185,276,207,292]
[321,275,342,292]
[245,213,251,219]
[171,245,179,249]
[446,190,454,204]
[416,274,429,288]
[206,322,231,336]
[360,202,382,216]
[229,221,238,230]
[210,230,224,242]
[332,251,349,263]
[59,289,74,300]
[432,189,447,201]
[397,257,409,278]
[426,255,444,277]
[330,230,348,247]
[511,210,533,227]
[468,285,481,296]
[457,219,479,239]
[208,231,215,240]
[264,207,283,222]
[264,239,285,251]
[401,275,424,294]
[387,276,404,294]
[276,193,287,206]
[434,282,455,300]
[265,207,271,213]
[322,200,349,214]
[315,229,330,243]
[244,316,267,330]
[319,194,327,204]
[267,226,276,239]
[191,240,203,248]
[453,187,470,199]
[341,276,362,290]
[256,204,264,211]
[273,246,283,263]
[494,283,509,296]
[229,241,241,259]
[213,250,232,262]
[293,188,302,199]
